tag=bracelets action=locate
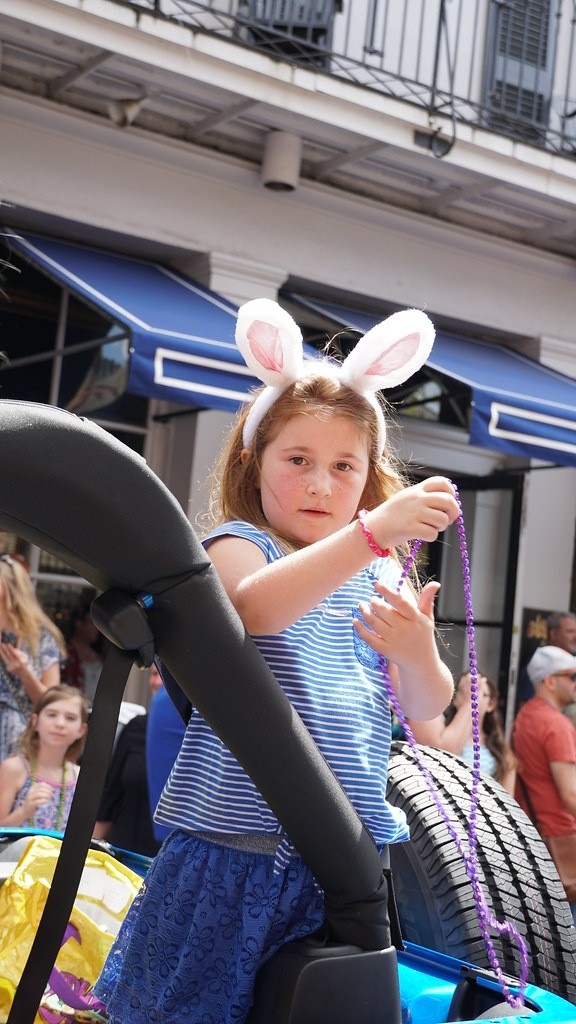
[358,509,390,558]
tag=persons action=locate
[513,646,576,901]
[548,610,576,721]
[393,669,516,798]
[94,374,455,1024]
[0,555,163,859]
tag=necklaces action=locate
[29,758,66,831]
[379,477,528,1006]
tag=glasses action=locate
[0,554,15,581]
[541,673,576,684]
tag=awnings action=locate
[285,290,575,470]
[0,227,321,412]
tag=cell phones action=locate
[1,629,19,648]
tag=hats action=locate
[526,646,576,685]
